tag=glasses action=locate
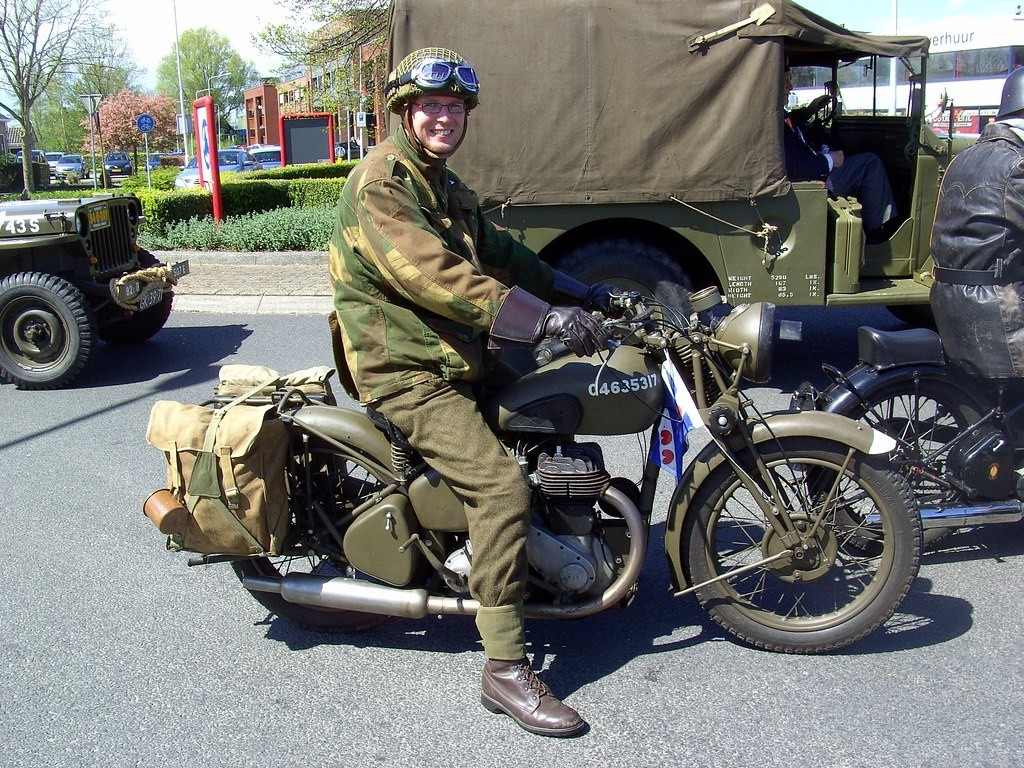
[412,101,468,116]
[410,58,480,94]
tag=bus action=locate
[789,6,1024,142]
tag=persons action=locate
[930,68,1024,380]
[784,63,897,240]
[328,48,623,737]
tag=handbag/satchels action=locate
[216,364,347,475]
[145,400,291,558]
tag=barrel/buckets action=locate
[828,194,863,294]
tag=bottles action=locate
[820,145,829,154]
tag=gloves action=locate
[486,285,612,358]
[549,271,624,319]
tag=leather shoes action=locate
[481,658,585,738]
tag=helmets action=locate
[995,65,1024,119]
[385,47,478,115]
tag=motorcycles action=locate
[137,281,925,658]
[795,323,1024,557]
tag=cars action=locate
[101,151,134,176]
[46,151,66,175]
[227,142,282,169]
[174,148,264,190]
[145,147,184,172]
[55,154,90,180]
[334,140,367,161]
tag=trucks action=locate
[0,189,192,391]
[380,1,981,332]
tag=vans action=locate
[15,149,46,164]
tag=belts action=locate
[935,267,1024,286]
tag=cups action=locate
[142,487,189,535]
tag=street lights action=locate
[194,84,223,99]
[208,71,232,96]
